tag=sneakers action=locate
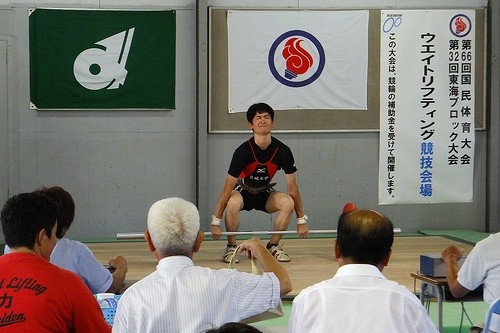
[222,243,239,263]
[265,244,292,263]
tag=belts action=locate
[238,179,277,194]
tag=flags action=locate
[28,8,176,111]
[227,11,369,114]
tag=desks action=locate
[410,272,483,333]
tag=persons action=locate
[211,103,309,263]
[441,232,500,333]
[0,193,113,333]
[199,321,262,333]
[111,197,293,333]
[287,209,439,333]
[4,186,128,297]
